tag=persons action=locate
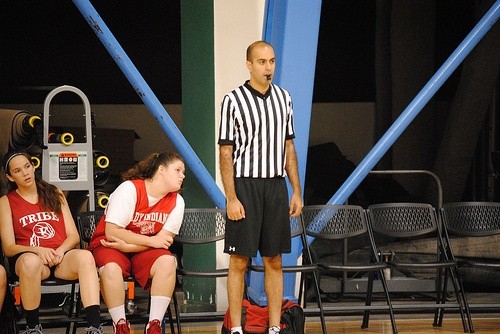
[0,265,8,312]
[85,152,185,334]
[218,40,303,334]
[0,149,107,334]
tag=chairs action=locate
[0,201,500,334]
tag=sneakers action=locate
[112,318,131,334]
[26,324,44,334]
[144,319,164,334]
[86,325,104,334]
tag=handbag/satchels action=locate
[221,294,305,334]
